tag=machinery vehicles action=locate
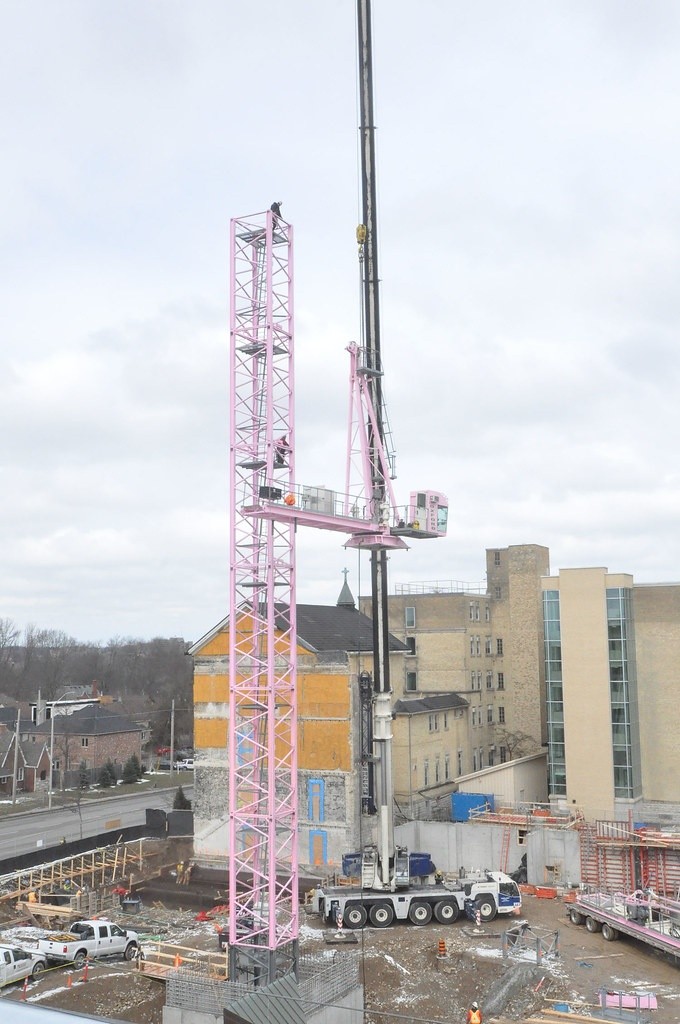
[302,0,522,982]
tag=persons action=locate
[82,884,86,891]
[76,889,83,895]
[434,871,443,884]
[60,837,66,845]
[276,434,292,464]
[65,878,69,890]
[183,866,193,885]
[270,200,284,231]
[27,889,38,903]
[466,1002,482,1024]
[570,800,580,821]
[176,861,185,883]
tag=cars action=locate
[160,761,176,770]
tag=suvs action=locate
[174,758,194,770]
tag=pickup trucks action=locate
[38,921,140,969]
[0,944,47,988]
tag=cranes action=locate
[146,212,449,992]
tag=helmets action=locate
[181,862,184,864]
[472,1002,478,1007]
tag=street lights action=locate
[49,691,76,811]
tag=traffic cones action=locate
[436,936,449,959]
[66,975,73,988]
